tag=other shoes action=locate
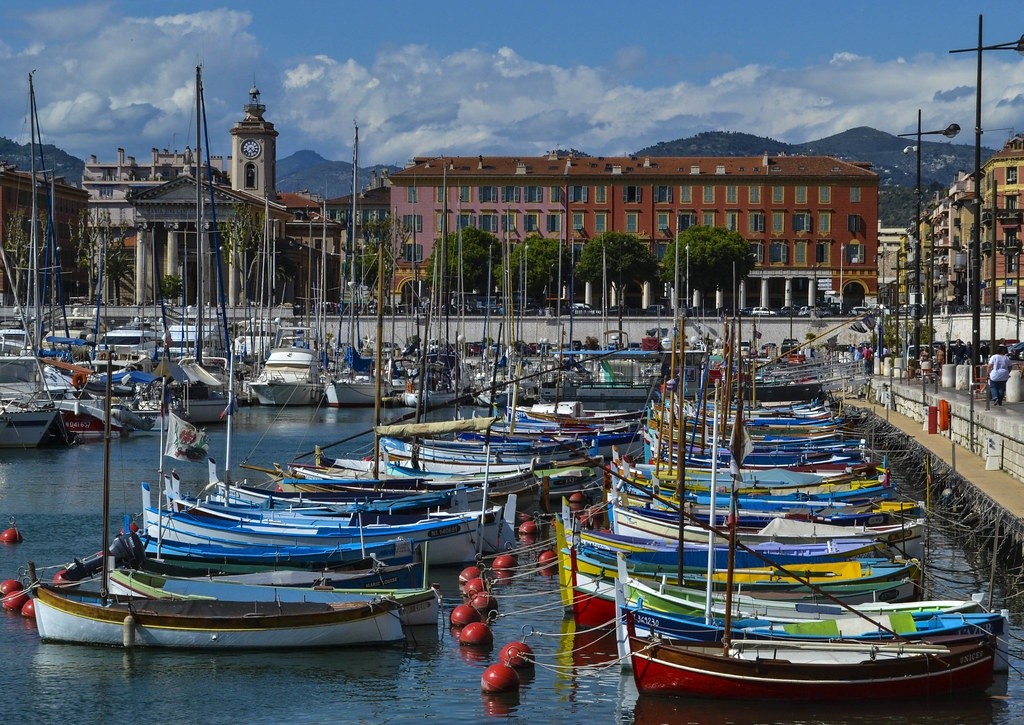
[993,399,999,406]
[999,399,1002,406]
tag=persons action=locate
[846,337,1012,406]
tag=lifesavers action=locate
[72,373,88,387]
[938,400,951,431]
[97,351,109,360]
[407,383,413,393]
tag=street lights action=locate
[945,14,1024,383]
[838,241,845,311]
[811,263,824,319]
[897,108,957,371]
[684,241,691,310]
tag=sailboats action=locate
[0,49,1003,704]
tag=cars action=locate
[797,304,812,317]
[893,304,926,317]
[777,305,802,318]
[609,304,625,315]
[573,303,592,311]
[648,304,666,311]
[849,306,866,317]
[747,307,776,318]
[740,307,752,316]
